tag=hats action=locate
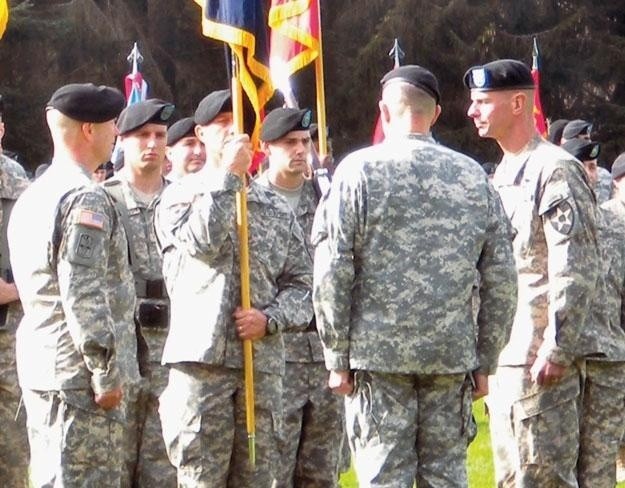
[480,161,500,177]
[308,122,334,143]
[163,116,199,149]
[610,151,625,180]
[113,97,176,137]
[193,88,234,126]
[96,160,114,170]
[562,119,594,141]
[2,150,20,162]
[45,82,128,124]
[462,59,536,93]
[379,63,441,107]
[257,106,314,142]
[564,140,602,163]
[547,118,572,146]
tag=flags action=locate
[195,0,321,166]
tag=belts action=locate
[0,302,13,330]
[131,296,173,333]
[304,314,319,331]
